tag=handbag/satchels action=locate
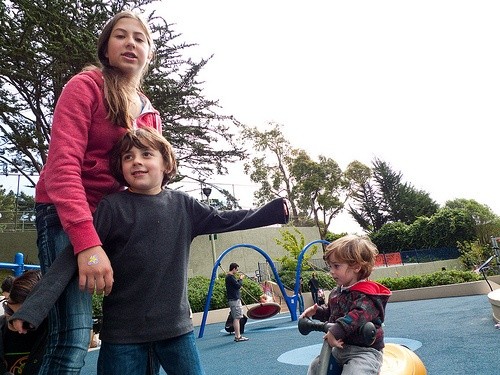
[317,289,323,297]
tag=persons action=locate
[298,234,391,375]
[8,126,290,375]
[0,269,48,375]
[308,272,319,305]
[225,311,247,335]
[318,286,325,301]
[0,275,16,317]
[225,263,249,342]
[30,10,163,375]
[294,277,305,315]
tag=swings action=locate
[217,260,282,320]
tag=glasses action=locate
[2,301,15,316]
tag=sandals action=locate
[234,335,249,342]
